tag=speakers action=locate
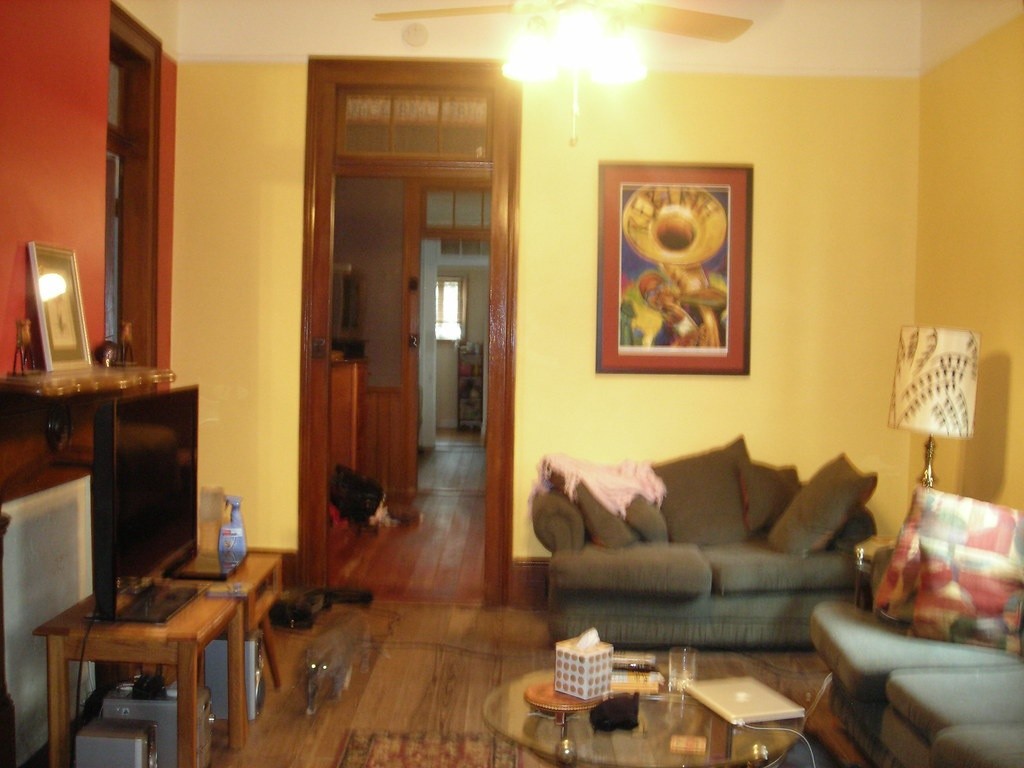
[204,629,266,720]
[75,719,157,768]
[101,679,215,768]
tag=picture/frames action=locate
[29,239,94,372]
[595,162,755,376]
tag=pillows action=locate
[737,463,800,537]
[871,483,1024,629]
[904,535,1024,658]
[766,454,878,558]
[542,458,640,549]
[626,497,670,545]
[650,434,755,547]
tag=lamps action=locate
[889,323,980,490]
[502,4,645,83]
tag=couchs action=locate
[811,598,1024,768]
[532,482,879,654]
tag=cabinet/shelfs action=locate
[457,346,483,431]
[326,360,380,549]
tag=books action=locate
[208,582,249,597]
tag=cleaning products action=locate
[218,494,248,563]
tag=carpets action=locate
[334,728,849,768]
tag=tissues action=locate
[554,627,614,700]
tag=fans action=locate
[370,1,753,44]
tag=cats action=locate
[296,608,391,715]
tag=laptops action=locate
[683,675,805,725]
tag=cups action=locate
[669,646,697,693]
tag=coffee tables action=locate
[479,658,805,768]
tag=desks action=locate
[33,555,284,768]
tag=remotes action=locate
[169,571,228,581]
[614,661,658,672]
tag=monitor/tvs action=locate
[82,384,214,625]
